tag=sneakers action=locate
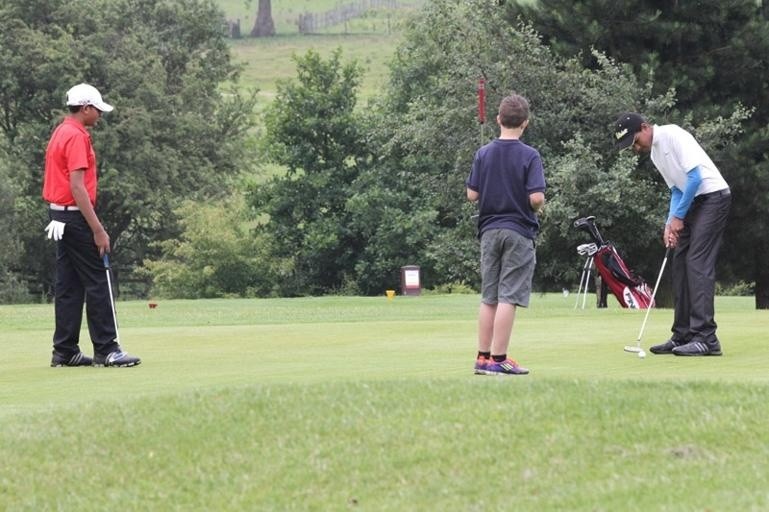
[92,349,142,368]
[474,355,489,374]
[671,339,722,356]
[650,342,683,354]
[485,356,529,376]
[50,350,93,367]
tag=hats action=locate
[613,112,646,154]
[66,82,115,112]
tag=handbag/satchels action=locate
[595,241,655,310]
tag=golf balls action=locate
[639,352,646,358]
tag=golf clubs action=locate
[624,241,670,352]
[574,244,598,309]
[574,216,604,247]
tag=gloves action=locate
[45,220,66,241]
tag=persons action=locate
[465,94,546,377]
[613,113,732,357]
[41,83,141,368]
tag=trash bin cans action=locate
[401,265,421,296]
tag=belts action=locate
[50,202,80,211]
